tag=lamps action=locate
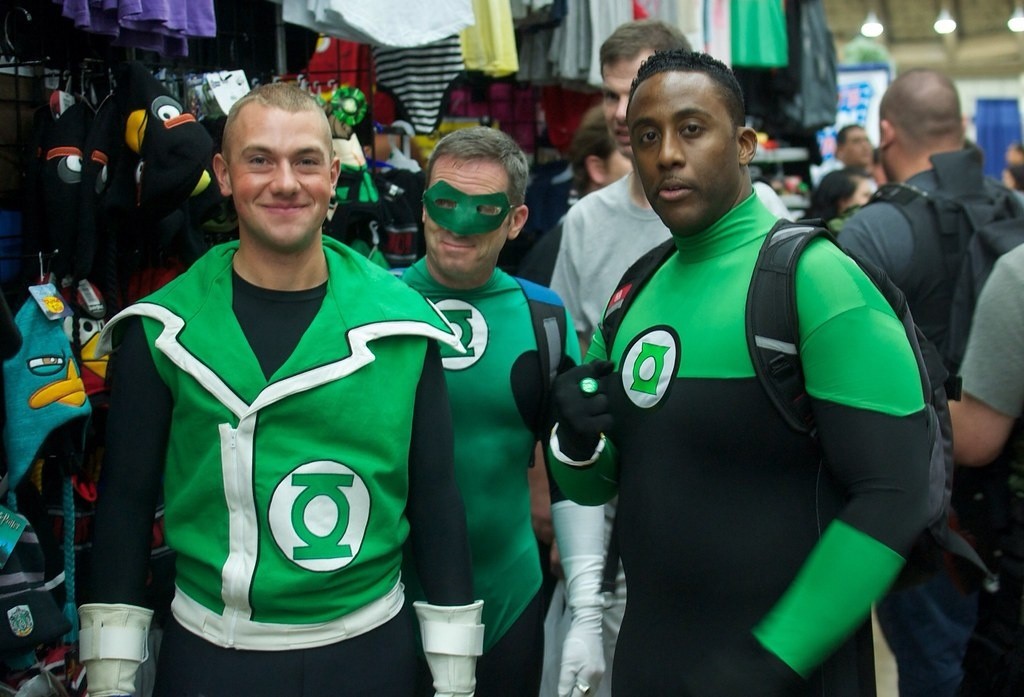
[861,11,885,36]
[934,8,957,35]
[1007,5,1024,32]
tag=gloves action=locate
[411,598,485,697]
[76,603,154,697]
[549,499,607,697]
[550,359,615,461]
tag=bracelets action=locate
[549,423,607,466]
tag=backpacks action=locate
[600,217,954,542]
[860,164,1024,375]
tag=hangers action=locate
[380,120,420,175]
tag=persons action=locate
[549,49,934,697]
[805,125,889,220]
[401,125,611,696]
[519,109,631,287]
[1000,141,1024,193]
[835,70,1024,697]
[79,84,486,697]
[948,243,1024,468]
[539,19,796,697]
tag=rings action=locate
[580,377,598,394]
